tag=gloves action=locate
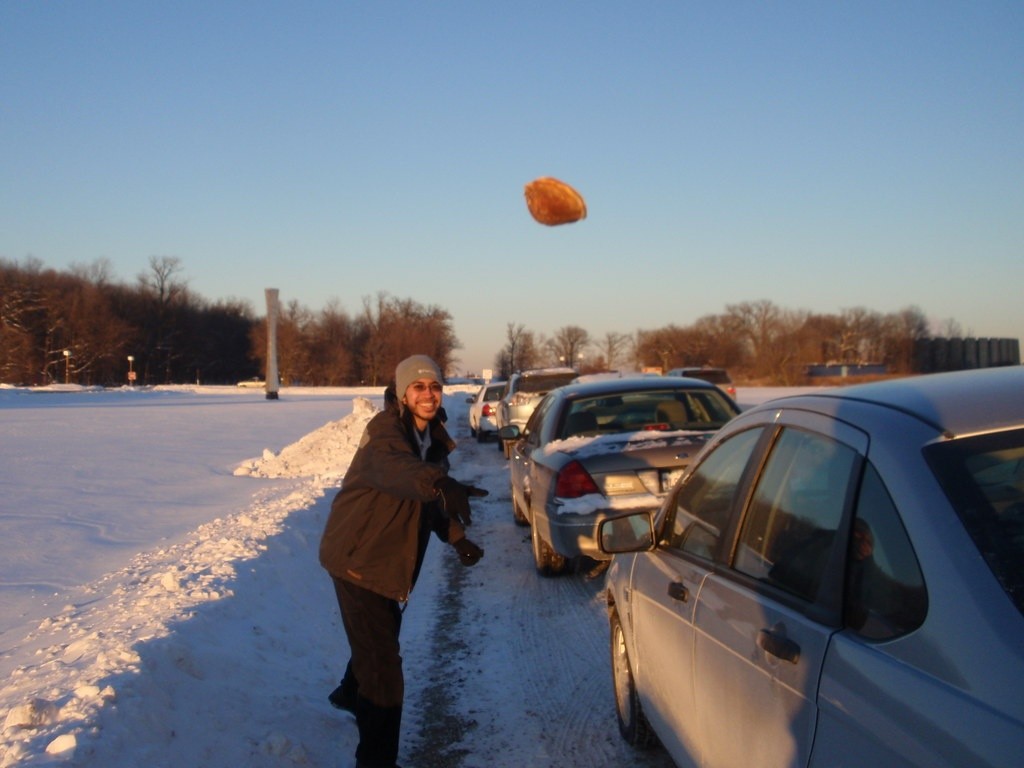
[433,474,488,529]
[453,537,483,567]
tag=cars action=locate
[237,375,268,390]
[465,380,508,444]
[488,366,574,463]
[597,363,1024,768]
[499,374,747,575]
[669,367,739,403]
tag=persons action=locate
[317,353,488,768]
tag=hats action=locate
[394,355,444,409]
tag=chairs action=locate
[563,412,599,435]
[654,403,686,423]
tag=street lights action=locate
[63,350,71,386]
[127,356,134,386]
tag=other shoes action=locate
[355,759,400,768]
[328,686,356,718]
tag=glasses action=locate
[409,381,442,391]
